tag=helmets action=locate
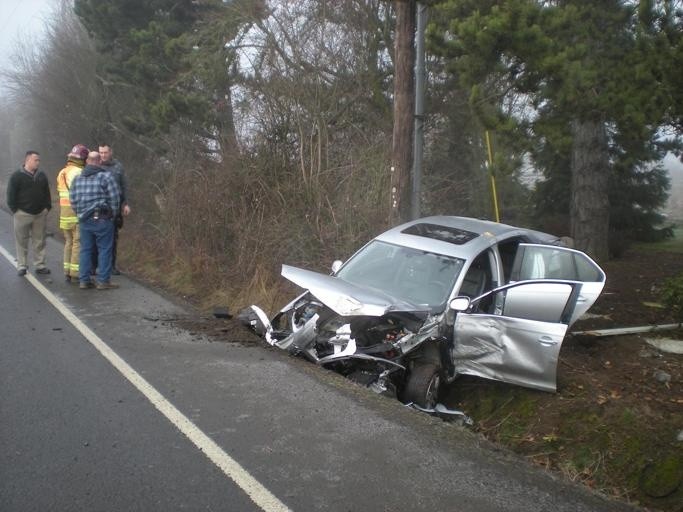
[68,144,89,159]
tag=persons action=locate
[6,151,51,277]
[56,145,89,284]
[69,151,121,289]
[90,142,131,275]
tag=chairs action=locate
[394,255,440,290]
[432,262,487,314]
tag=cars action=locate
[251,209,607,411]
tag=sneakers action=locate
[97,282,118,290]
[111,267,121,275]
[36,268,49,274]
[64,269,97,289]
[17,270,26,276]
